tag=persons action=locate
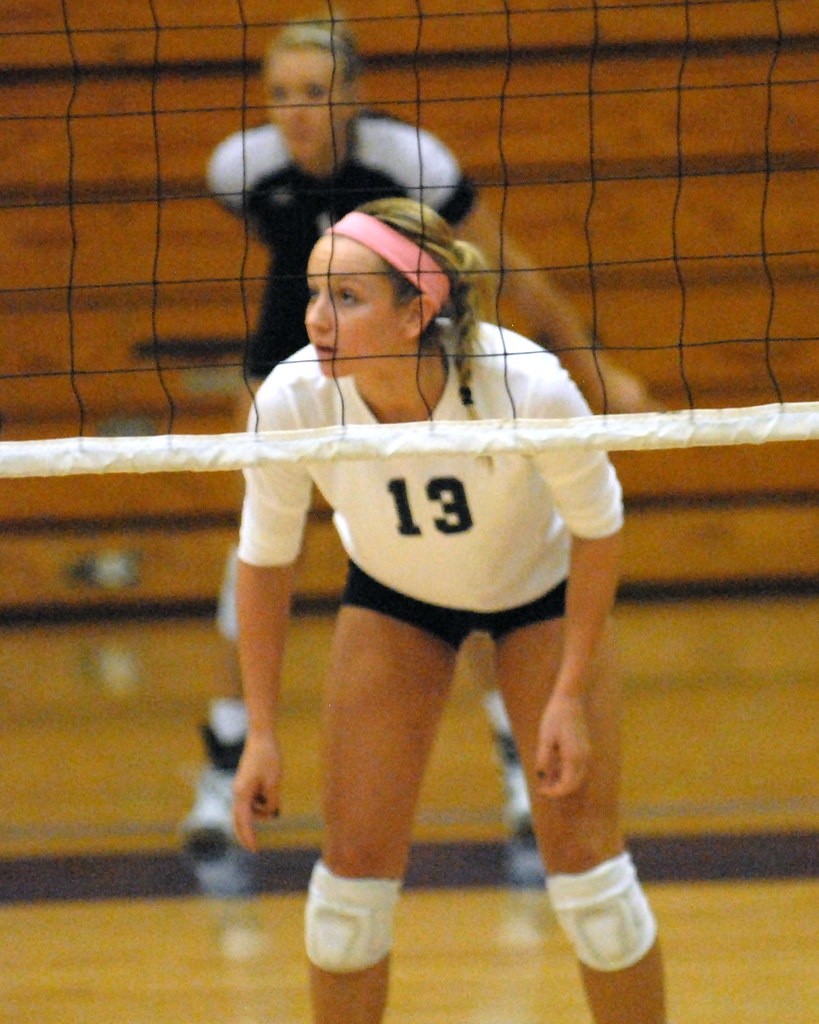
[177,25,654,880]
[234,196,664,1024]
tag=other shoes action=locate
[483,697,535,831]
[183,697,258,843]
